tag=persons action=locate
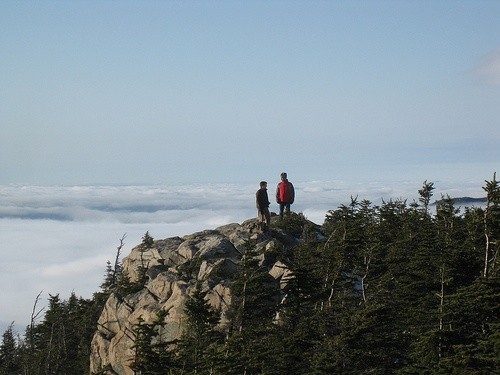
[275,172,295,221]
[255,181,271,225]
